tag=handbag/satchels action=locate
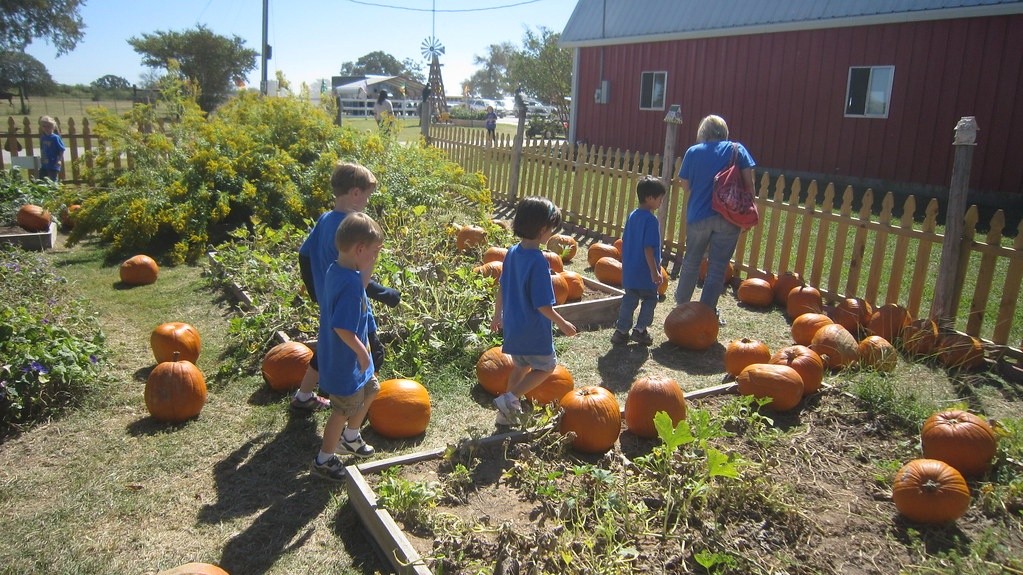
[711,142,758,228]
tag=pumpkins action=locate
[16,204,51,232]
[158,563,229,575]
[893,458,970,524]
[920,410,996,475]
[145,351,207,421]
[261,341,314,392]
[60,204,81,227]
[369,378,431,438]
[150,322,201,365]
[477,344,687,453]
[445,219,669,305]
[120,255,159,285]
[665,257,985,410]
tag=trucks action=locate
[504,97,515,114]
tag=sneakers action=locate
[334,434,374,457]
[630,329,652,344]
[309,455,346,480]
[493,393,517,425]
[290,391,331,411]
[611,329,631,344]
[495,412,520,425]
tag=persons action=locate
[309,213,384,482]
[490,195,577,427]
[484,106,496,142]
[611,176,665,347]
[373,89,395,148]
[290,162,401,411]
[38,115,65,182]
[677,114,756,326]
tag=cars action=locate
[513,100,548,119]
[470,99,505,118]
[446,99,465,111]
[545,105,557,115]
